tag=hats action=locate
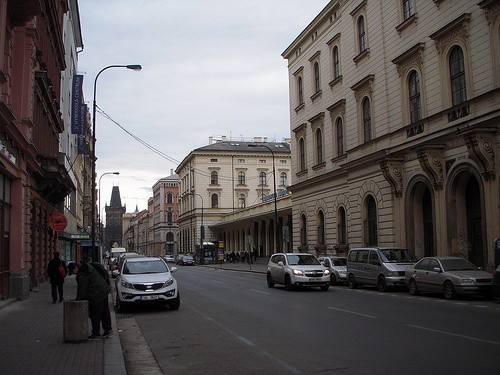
[67,262,75,276]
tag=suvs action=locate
[112,257,181,311]
[266,253,331,293]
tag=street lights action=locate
[90,64,143,264]
[247,144,280,254]
[98,171,119,244]
[186,193,203,265]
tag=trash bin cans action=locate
[62,298,90,343]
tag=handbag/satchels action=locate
[60,260,66,277]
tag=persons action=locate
[46,251,67,304]
[225,246,257,264]
[67,261,114,341]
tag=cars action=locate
[317,257,350,286]
[109,252,145,278]
[405,257,494,300]
[163,254,196,266]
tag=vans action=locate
[345,247,420,293]
[109,247,126,265]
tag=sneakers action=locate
[100,330,114,338]
[88,334,101,340]
